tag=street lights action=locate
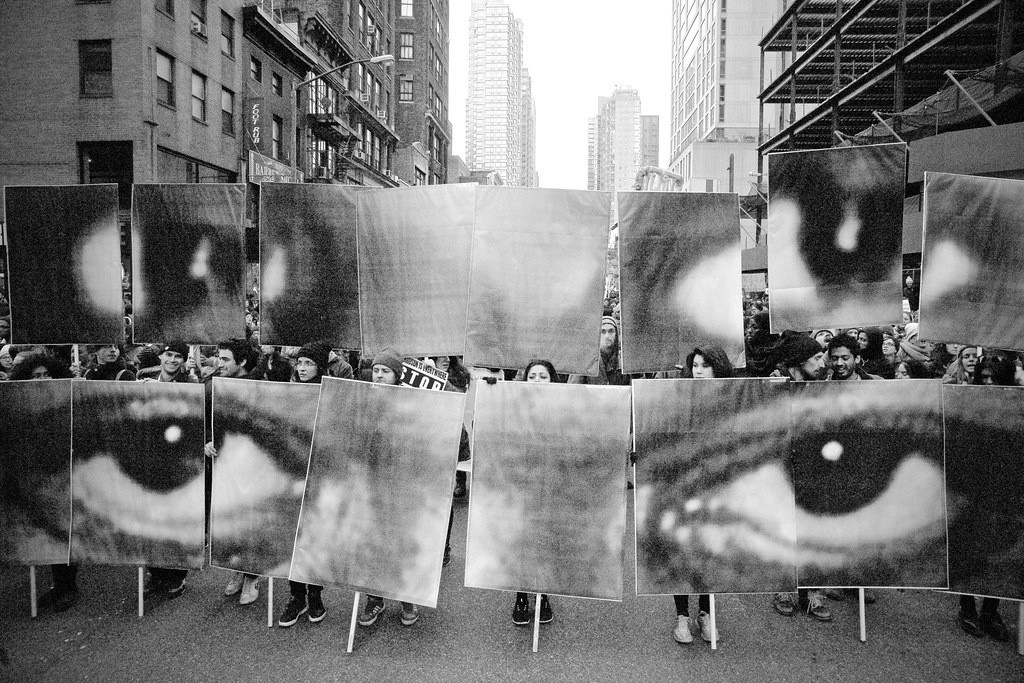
[289,55,397,184]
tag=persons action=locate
[137,342,199,596]
[358,346,419,626]
[481,360,560,625]
[770,334,831,621]
[673,344,737,645]
[279,340,334,626]
[8,355,78,612]
[203,339,261,605]
[0,287,1024,497]
[820,334,887,602]
[957,352,1010,640]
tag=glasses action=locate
[1,356,12,361]
[979,354,1005,364]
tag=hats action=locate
[158,340,187,361]
[879,325,894,336]
[752,313,769,333]
[779,330,822,367]
[905,322,919,340]
[956,345,969,358]
[296,341,332,369]
[370,346,404,378]
[0,344,10,357]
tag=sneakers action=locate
[672,616,694,644]
[534,595,553,623]
[399,603,418,625]
[225,574,259,605]
[278,597,308,626]
[696,611,720,642]
[958,612,985,635]
[512,599,531,624]
[774,592,794,614]
[359,599,386,626]
[308,592,325,621]
[802,591,833,621]
[980,614,1010,641]
[143,573,187,598]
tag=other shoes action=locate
[443,551,451,566]
[453,482,466,497]
[40,584,79,610]
